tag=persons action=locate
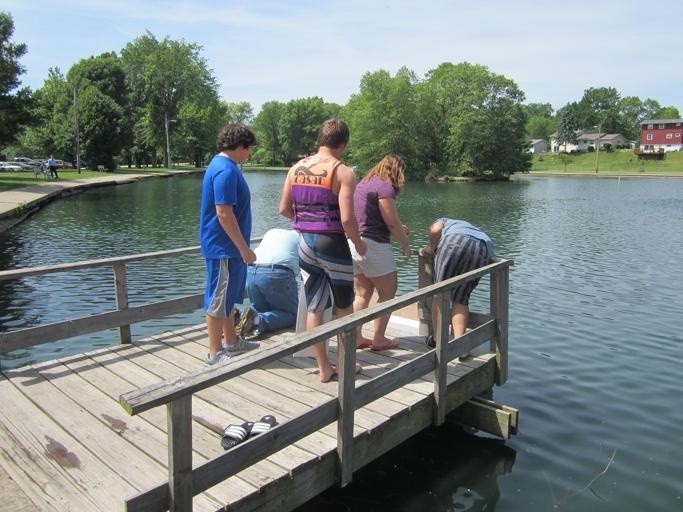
[421,217,493,361]
[200,123,260,365]
[47,155,59,179]
[345,154,410,351]
[219,228,301,341]
[278,119,368,383]
[40,161,51,180]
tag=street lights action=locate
[590,124,601,173]
[164,119,177,168]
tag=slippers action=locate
[355,339,373,349]
[369,339,399,350]
[221,421,255,450]
[249,415,276,439]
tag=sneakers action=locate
[204,347,234,366]
[458,353,471,361]
[222,335,260,355]
[221,307,257,339]
[425,336,436,347]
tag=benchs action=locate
[96,164,109,175]
[32,167,55,181]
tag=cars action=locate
[0,155,85,172]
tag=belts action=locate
[248,263,295,275]
